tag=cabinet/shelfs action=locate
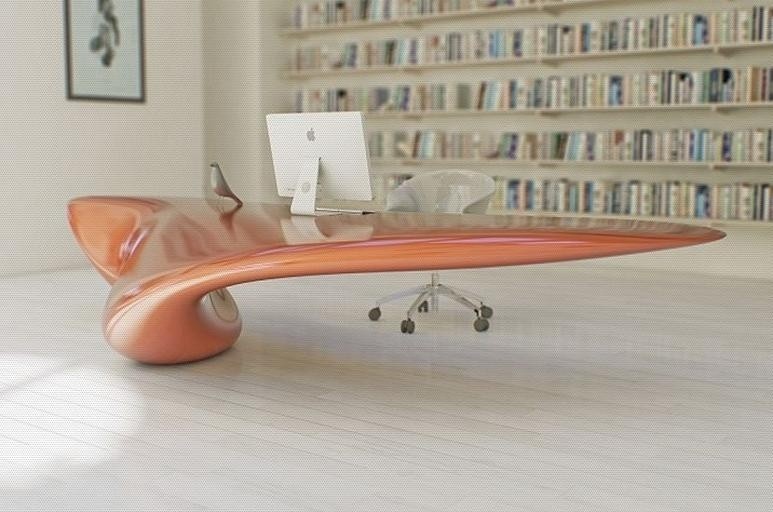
[280,2,773,230]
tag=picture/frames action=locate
[64,1,146,101]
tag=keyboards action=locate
[316,208,377,215]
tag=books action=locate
[291,0,773,222]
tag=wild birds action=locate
[209,160,244,207]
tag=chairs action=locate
[368,168,496,333]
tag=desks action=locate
[66,196,726,367]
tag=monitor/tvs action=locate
[266,111,375,217]
[279,217,374,246]
